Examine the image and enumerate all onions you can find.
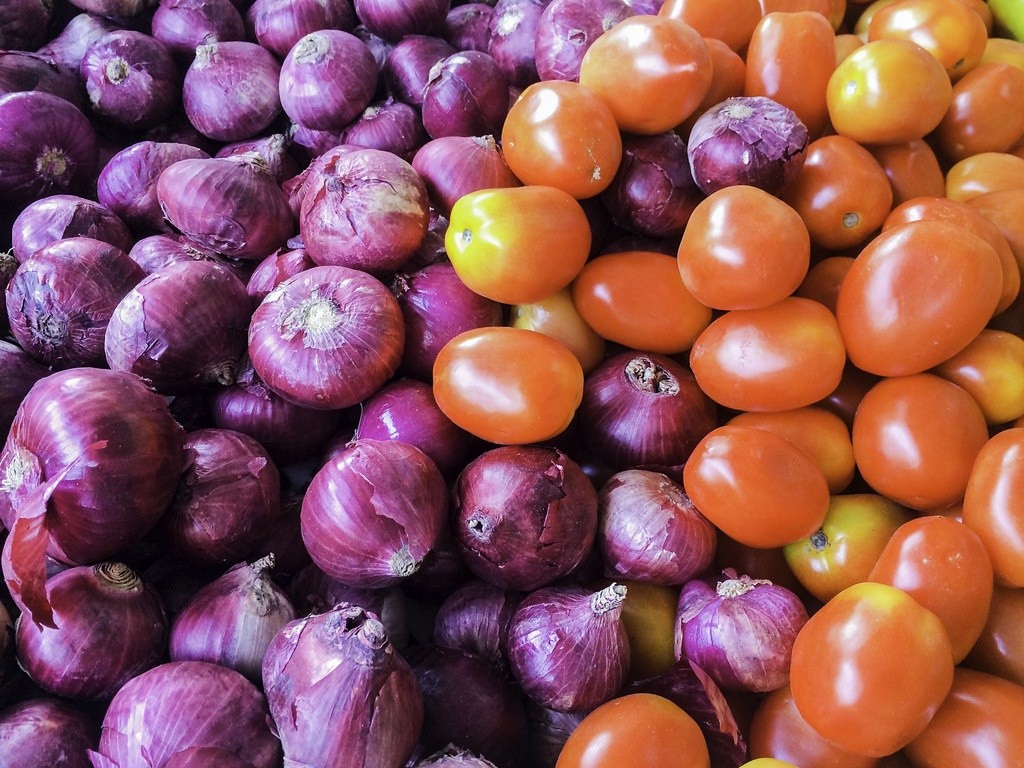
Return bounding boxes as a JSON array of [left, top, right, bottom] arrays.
[[0, 0, 811, 768]]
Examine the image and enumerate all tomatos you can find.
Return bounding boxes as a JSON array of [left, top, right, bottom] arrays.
[[431, 0, 1024, 768]]
